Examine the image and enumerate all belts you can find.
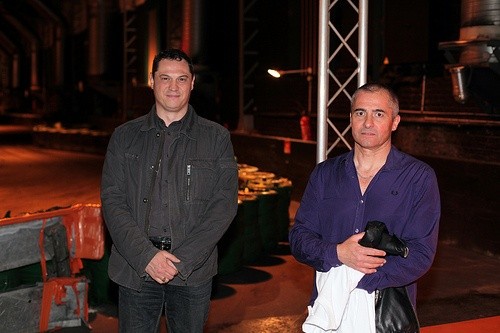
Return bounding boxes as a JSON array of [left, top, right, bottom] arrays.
[[149, 240, 172, 252]]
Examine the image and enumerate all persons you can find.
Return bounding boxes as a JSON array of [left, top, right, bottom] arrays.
[[289, 81, 441, 332], [99, 48, 240, 333]]
